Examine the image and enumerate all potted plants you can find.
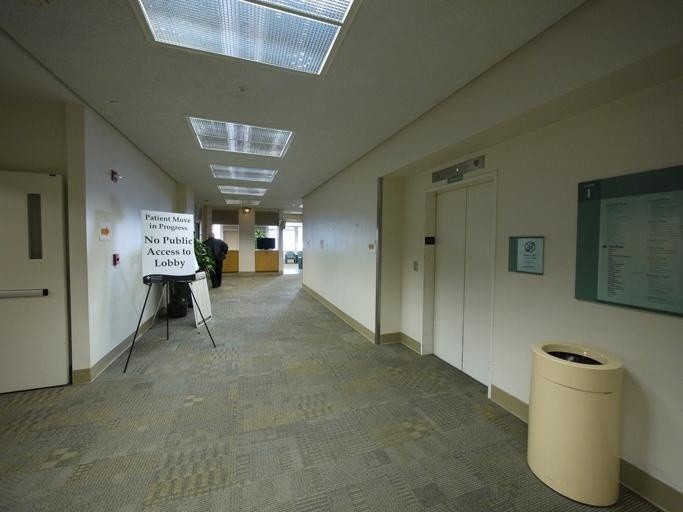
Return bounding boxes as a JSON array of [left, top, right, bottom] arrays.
[[168, 237, 215, 318]]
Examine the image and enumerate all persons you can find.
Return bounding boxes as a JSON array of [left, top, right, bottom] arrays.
[[200, 233, 228, 287]]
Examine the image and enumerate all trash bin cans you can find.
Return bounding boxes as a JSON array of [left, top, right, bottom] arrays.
[[527, 341, 623, 506]]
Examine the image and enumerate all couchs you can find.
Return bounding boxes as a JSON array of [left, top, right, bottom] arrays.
[[284, 251, 296, 264], [295, 250, 302, 263]]
